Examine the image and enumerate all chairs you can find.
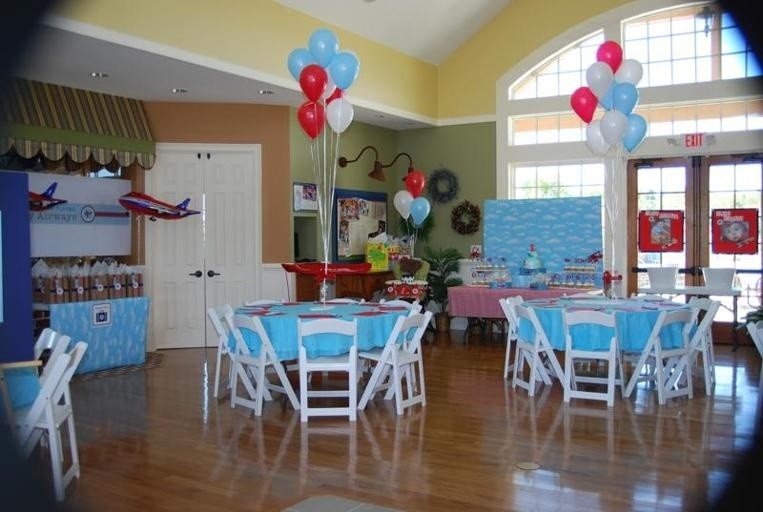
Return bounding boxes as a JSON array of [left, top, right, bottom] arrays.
[[0, 325, 89, 503], [207, 256, 432, 423], [746, 320, 763, 395], [498, 294, 721, 407]]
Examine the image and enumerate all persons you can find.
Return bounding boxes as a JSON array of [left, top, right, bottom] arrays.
[[338, 198, 369, 243], [302, 184, 316, 201]]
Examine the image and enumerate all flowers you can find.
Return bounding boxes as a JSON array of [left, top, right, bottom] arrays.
[[449, 199, 480, 234]]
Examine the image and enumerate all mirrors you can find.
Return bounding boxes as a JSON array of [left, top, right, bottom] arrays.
[[293, 214, 320, 263]]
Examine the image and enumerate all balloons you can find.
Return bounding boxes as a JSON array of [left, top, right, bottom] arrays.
[[393, 190, 414, 219], [405, 170, 425, 198], [410, 197, 430, 225], [569, 41, 648, 158], [286, 27, 360, 139]]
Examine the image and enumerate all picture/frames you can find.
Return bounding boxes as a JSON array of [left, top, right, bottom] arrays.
[[712, 209, 758, 255], [638, 210, 684, 253]]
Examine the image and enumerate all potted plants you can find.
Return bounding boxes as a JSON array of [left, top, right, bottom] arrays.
[[422, 245, 467, 332]]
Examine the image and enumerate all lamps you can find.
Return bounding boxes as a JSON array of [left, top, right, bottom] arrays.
[[381, 153, 414, 181], [339, 145, 385, 181]]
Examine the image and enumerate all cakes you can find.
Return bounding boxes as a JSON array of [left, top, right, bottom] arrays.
[[522, 244, 542, 269]]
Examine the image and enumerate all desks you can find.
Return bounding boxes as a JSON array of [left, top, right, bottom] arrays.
[[447, 285, 603, 345], [639, 286, 742, 352]]
